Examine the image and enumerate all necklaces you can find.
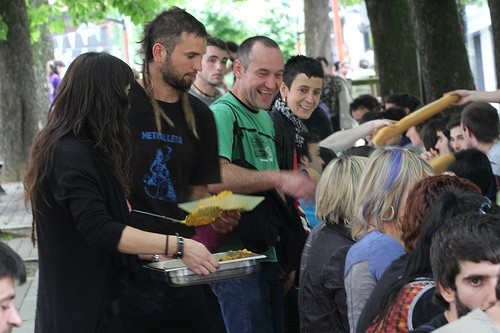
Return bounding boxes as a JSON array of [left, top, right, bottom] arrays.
[[192, 81, 217, 99]]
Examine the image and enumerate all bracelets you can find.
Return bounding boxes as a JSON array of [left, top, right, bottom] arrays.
[[164, 234, 169, 255], [172, 236, 184, 259], [152, 253, 159, 262]]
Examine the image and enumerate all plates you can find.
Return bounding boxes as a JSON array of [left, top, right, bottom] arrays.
[[178, 194, 265, 214]]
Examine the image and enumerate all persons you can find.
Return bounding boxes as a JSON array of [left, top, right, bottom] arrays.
[[190, 38, 239, 106], [22, 52, 218, 333], [46, 60, 65, 103], [208, 36, 316, 333], [129, 5, 243, 333], [267, 55, 500, 333], [0, 242, 27, 333]]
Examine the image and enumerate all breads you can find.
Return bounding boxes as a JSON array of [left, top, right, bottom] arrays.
[[373, 95, 457, 144], [426, 152, 455, 172]]
